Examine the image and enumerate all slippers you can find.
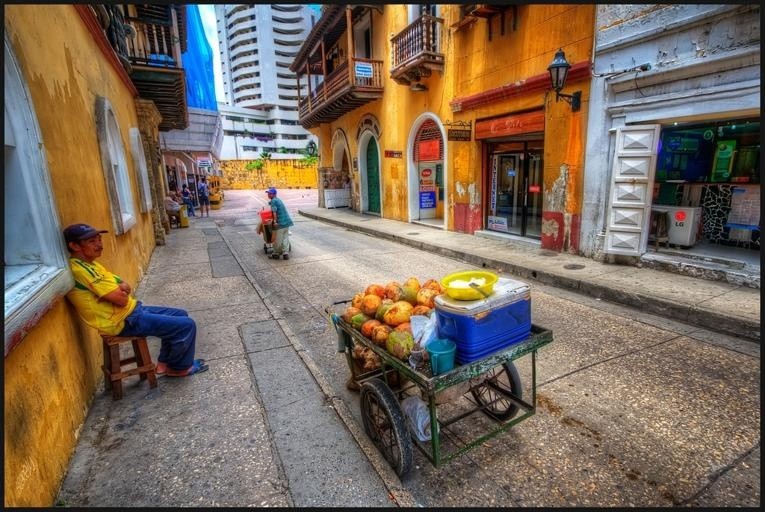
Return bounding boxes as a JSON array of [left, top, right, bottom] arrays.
[[198, 183, 207, 200]]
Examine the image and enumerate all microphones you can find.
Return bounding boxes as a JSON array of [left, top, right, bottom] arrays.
[[165, 208, 179, 228], [100, 330, 158, 402]]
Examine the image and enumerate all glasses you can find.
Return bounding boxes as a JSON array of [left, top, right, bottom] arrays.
[[409, 76, 427, 91], [545, 46, 581, 112]]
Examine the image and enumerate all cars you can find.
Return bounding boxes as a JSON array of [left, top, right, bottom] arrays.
[[427, 339, 458, 376], [409, 346, 424, 367]]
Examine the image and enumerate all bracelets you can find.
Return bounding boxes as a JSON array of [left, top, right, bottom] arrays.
[[440, 271, 498, 301]]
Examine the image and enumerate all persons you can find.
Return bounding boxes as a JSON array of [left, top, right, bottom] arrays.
[[63, 224, 210, 378], [165, 176, 210, 223], [265, 188, 294, 260]]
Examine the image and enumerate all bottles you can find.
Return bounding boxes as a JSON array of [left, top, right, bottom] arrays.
[[264, 187, 278, 195], [62, 222, 111, 244]]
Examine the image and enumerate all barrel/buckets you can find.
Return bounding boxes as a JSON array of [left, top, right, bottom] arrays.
[[154, 357, 210, 378]]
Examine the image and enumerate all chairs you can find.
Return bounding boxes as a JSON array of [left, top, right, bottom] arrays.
[[268, 254, 280, 260], [282, 254, 289, 260]]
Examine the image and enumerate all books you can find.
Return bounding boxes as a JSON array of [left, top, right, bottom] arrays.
[[432, 276, 533, 364]]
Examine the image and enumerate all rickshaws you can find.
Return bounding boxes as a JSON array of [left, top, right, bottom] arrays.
[[343, 277, 441, 358]]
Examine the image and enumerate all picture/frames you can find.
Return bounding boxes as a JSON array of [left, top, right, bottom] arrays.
[[724, 223, 759, 249]]
